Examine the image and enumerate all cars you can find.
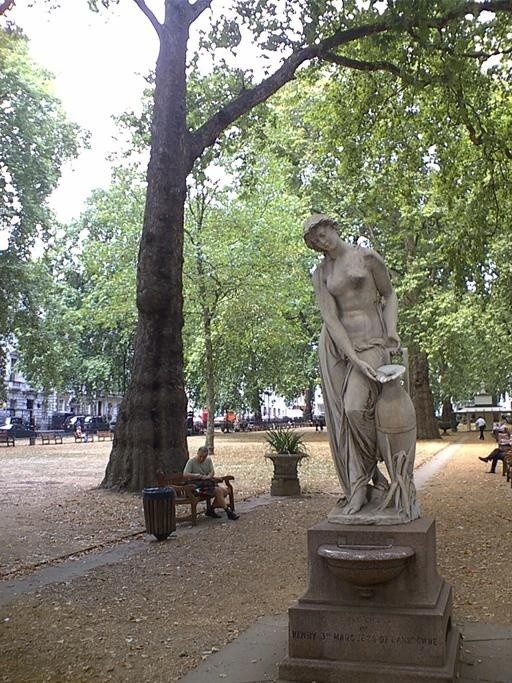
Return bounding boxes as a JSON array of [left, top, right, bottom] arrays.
[[0, 409, 109, 438]]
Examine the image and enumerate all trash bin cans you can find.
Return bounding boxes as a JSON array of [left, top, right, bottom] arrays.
[[30, 438, 35, 445], [142, 487, 176, 542]]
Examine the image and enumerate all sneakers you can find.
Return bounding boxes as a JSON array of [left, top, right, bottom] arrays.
[[205, 510, 222, 519], [478, 456, 488, 462], [485, 470, 496, 473], [227, 512, 241, 520]]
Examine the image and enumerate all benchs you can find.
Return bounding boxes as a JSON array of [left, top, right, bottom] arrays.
[[0, 430, 113, 448], [154, 469, 236, 524]]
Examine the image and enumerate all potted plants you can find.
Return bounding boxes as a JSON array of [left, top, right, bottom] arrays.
[[264, 427, 310, 496]]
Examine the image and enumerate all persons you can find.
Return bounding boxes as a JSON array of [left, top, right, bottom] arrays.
[[314, 418, 320, 432], [474, 414, 512, 473], [287, 419, 292, 425], [75, 417, 83, 431], [184, 446, 240, 520], [77, 425, 88, 442], [318, 415, 324, 430], [302, 213, 400, 517]]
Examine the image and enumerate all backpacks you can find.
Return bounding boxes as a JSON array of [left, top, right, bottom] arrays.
[[183, 479, 216, 498]]
